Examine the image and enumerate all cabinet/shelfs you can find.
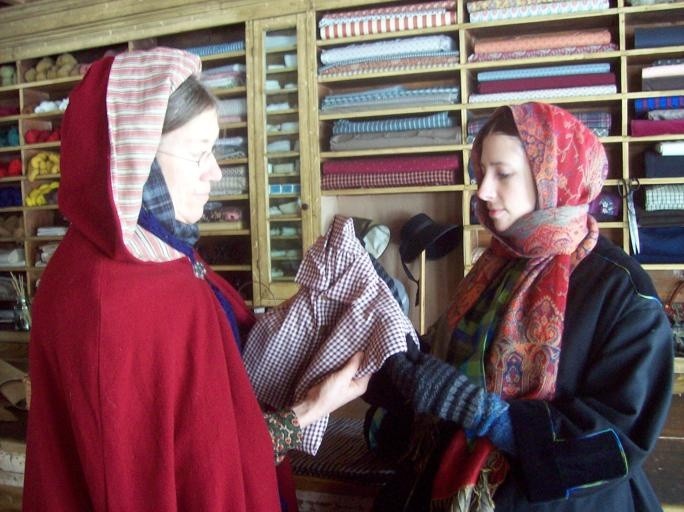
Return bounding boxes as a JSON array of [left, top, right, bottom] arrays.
[[619, 1, 683, 269], [458, 2, 627, 270], [20, 26, 133, 331], [250, 1, 318, 301], [316, 3, 466, 382], [129, 13, 256, 318], [2, 35, 30, 337]]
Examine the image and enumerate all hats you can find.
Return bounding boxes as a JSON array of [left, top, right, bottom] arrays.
[[399, 214, 459, 262]]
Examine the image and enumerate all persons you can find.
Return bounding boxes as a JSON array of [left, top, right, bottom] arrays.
[[361, 102, 674, 512], [20, 46, 371, 512]]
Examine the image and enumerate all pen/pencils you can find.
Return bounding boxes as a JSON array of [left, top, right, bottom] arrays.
[[8, 271, 32, 328]]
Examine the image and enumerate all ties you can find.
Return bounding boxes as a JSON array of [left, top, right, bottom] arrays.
[[158, 143, 216, 167]]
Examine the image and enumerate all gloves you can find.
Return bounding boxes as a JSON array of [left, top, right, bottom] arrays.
[[366, 333, 485, 429]]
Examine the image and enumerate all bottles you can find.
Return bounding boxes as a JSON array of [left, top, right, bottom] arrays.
[[14, 297, 31, 332]]
